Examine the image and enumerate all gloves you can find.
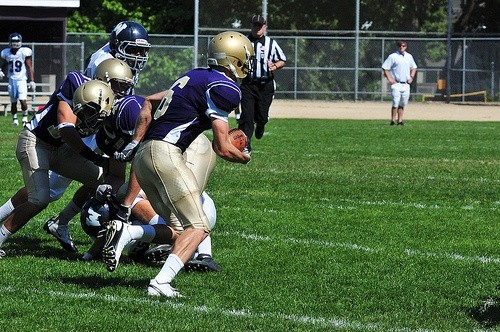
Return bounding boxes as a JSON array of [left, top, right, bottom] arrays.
[[114, 141, 139, 163], [28, 81, 36, 89], [0, 68, 5, 80]]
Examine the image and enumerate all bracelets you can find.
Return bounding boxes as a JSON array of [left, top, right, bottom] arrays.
[[411, 76, 414, 80], [275, 64, 277, 69]]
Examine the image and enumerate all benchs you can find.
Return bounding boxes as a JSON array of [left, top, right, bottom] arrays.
[[0, 101, 47, 117]]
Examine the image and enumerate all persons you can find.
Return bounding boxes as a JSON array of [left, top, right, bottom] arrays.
[[0, 33, 34, 126], [237, 15, 287, 153], [383, 41, 417, 126], [0, 22, 252, 297]]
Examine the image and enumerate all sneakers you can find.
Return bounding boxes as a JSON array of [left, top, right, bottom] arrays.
[[184, 254, 222, 273], [147, 279, 185, 297], [102, 220, 131, 272], [147, 244, 175, 265], [43, 215, 79, 253], [128, 240, 149, 256]]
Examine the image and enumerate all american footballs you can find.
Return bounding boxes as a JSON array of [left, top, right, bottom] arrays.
[[211, 128, 250, 153]]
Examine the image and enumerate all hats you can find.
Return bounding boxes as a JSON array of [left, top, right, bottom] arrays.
[[250, 14, 266, 25]]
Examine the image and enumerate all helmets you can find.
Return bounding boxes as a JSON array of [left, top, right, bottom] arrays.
[[109, 21, 148, 58], [9, 33, 22, 49], [73, 79, 115, 116], [80, 197, 117, 239], [207, 31, 254, 79], [93, 58, 134, 86]]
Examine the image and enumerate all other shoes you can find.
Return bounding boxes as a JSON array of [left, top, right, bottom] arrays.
[[254, 124, 264, 139], [242, 143, 252, 155], [22, 116, 27, 122], [390, 120, 396, 125], [398, 121, 404, 125], [13, 119, 19, 126]]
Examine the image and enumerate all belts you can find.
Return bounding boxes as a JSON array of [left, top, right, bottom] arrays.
[[396, 80, 405, 84]]
